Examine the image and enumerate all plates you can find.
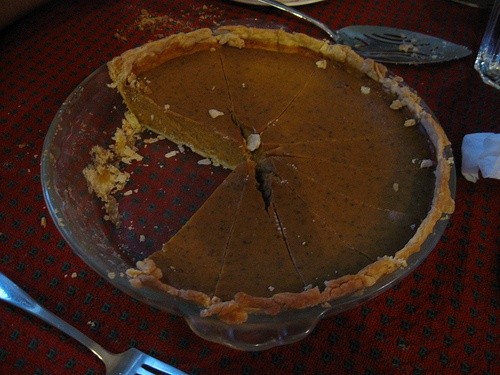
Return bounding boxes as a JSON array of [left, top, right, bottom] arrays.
[[40, 25, 457, 352]]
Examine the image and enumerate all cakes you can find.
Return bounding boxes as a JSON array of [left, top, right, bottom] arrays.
[[105, 25, 456, 324]]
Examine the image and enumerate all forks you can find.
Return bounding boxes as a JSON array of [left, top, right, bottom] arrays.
[[1, 272, 187, 375]]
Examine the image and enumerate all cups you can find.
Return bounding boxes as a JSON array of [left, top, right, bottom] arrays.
[[474, 0, 500, 86]]
